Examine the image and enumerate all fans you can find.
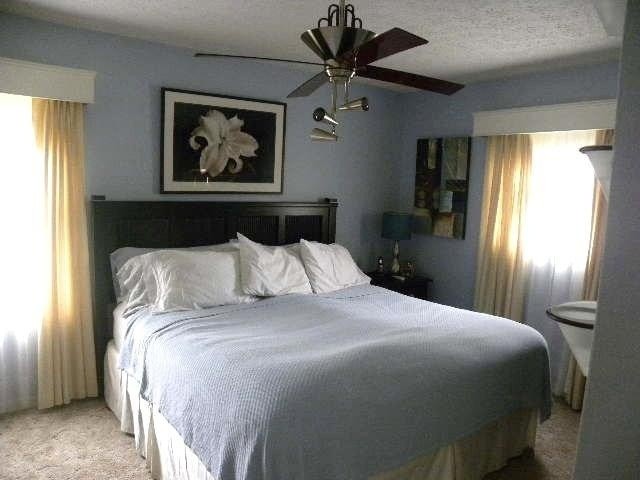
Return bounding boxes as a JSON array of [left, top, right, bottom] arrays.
[[192, 1, 466, 99]]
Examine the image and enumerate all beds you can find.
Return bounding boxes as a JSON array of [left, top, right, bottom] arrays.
[[87, 194, 550, 480]]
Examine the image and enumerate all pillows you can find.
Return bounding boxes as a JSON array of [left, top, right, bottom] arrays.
[[148, 248, 249, 315], [298, 237, 373, 296], [236, 231, 313, 296]]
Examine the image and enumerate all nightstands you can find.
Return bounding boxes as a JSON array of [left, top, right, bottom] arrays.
[[364, 272, 433, 301]]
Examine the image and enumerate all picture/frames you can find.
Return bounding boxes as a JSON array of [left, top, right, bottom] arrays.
[[158, 86, 288, 196], [413, 136, 473, 242]]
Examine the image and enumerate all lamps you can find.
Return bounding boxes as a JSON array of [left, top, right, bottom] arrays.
[[381, 211, 416, 279], [300, 67, 379, 143]]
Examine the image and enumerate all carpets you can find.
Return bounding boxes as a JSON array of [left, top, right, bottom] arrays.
[[0, 394, 157, 480], [484, 390, 583, 480]]
